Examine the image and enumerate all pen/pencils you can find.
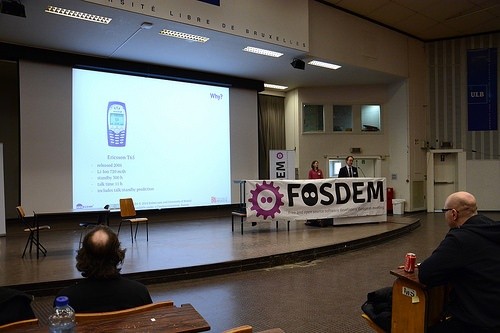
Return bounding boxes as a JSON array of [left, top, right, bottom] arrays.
[[398, 264, 418, 268]]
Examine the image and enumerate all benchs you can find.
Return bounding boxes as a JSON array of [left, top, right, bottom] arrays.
[[0, 300, 175, 329]]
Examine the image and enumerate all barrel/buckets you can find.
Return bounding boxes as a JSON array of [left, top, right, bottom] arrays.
[[392, 199, 406, 214]]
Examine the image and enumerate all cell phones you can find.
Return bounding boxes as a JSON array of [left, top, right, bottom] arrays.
[[107, 101, 127, 147]]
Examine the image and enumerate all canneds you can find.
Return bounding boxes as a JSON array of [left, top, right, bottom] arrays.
[[404, 253, 416, 273]]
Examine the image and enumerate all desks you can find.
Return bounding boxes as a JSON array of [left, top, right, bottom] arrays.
[[29, 209, 110, 260], [232, 178, 387, 235], [390, 266, 454, 333], [0, 303, 211, 333]]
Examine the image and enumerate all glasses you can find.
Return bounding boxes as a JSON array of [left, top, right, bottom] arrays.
[[442, 208, 459, 213]]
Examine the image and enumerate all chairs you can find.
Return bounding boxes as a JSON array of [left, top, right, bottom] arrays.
[[79, 205, 111, 250], [117, 198, 149, 243], [15, 206, 51, 261]]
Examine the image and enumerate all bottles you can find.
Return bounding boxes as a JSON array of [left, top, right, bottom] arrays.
[[48, 296, 75, 333]]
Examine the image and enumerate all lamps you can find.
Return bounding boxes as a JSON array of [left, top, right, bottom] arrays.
[[0, 0, 26, 18], [290, 57, 306, 70]]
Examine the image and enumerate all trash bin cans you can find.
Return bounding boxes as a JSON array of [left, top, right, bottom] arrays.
[[392, 198, 405, 214]]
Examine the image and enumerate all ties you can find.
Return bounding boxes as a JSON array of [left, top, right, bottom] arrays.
[[349, 166, 352, 177]]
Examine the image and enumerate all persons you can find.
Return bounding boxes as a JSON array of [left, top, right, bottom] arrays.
[[53, 225, 153, 313], [338, 156, 358, 177], [309, 160, 324, 179], [417, 192, 500, 333]]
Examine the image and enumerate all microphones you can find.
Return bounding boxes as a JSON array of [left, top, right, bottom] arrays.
[[358, 167, 365, 177]]
